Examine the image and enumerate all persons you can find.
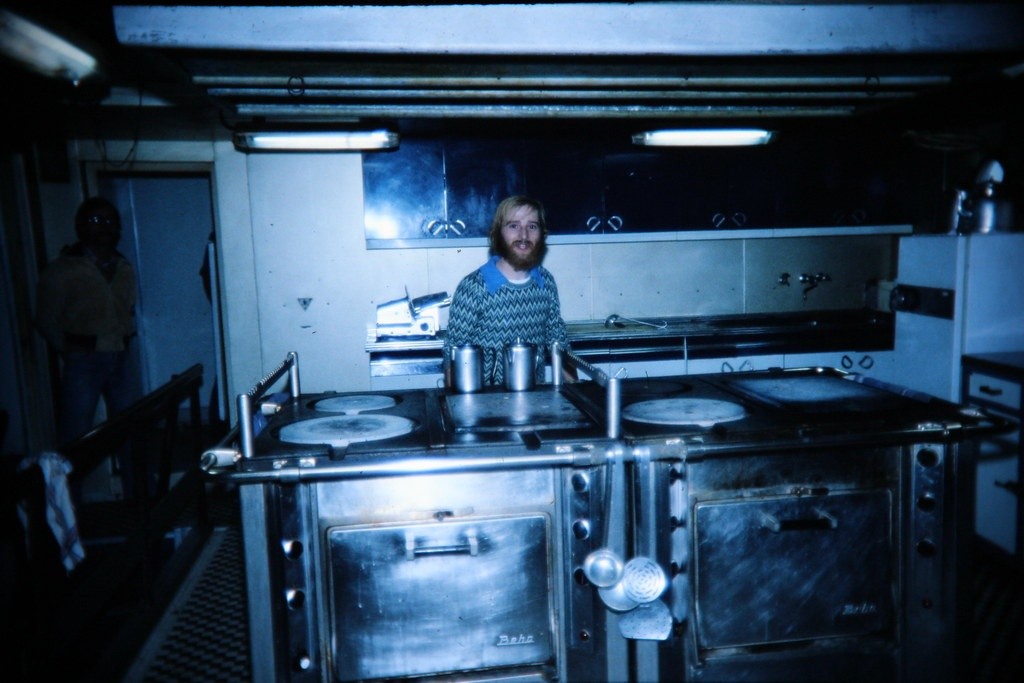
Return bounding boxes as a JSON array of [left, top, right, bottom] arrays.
[[36, 196, 153, 493], [444, 195, 580, 386]]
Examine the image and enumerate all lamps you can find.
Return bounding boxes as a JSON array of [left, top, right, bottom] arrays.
[[629, 124, 773, 147], [233, 128, 399, 152]]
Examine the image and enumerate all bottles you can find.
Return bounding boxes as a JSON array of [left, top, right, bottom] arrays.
[[978, 183, 995, 234]]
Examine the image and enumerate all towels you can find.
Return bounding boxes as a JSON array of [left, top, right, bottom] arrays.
[[40, 451, 86, 576]]
